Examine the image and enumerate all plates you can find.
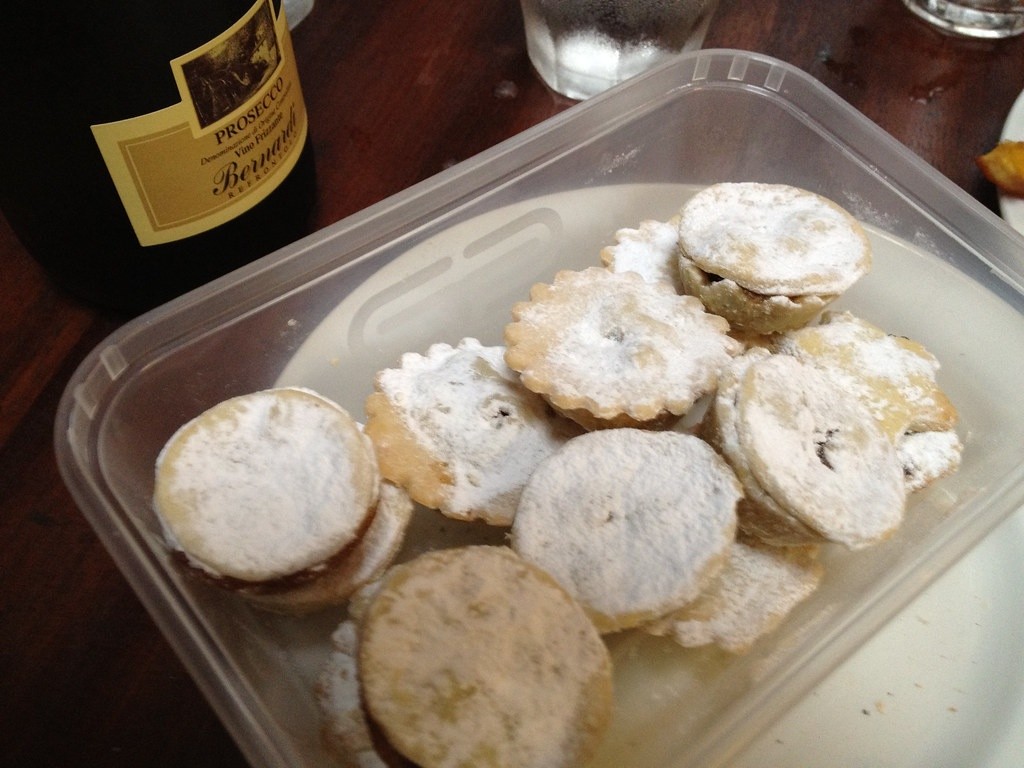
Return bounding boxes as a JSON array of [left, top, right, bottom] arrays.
[[264, 178, 1024, 768], [998, 86, 1024, 233]]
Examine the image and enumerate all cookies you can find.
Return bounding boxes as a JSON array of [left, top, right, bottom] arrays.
[[151, 181, 963, 768]]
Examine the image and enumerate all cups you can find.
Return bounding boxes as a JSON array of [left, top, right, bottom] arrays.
[[519, 0, 715, 103]]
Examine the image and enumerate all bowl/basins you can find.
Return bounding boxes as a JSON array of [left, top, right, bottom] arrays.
[[56, 43, 1024, 768]]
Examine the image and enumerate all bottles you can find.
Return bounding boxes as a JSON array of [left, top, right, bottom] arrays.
[[0, 0, 319, 315]]
